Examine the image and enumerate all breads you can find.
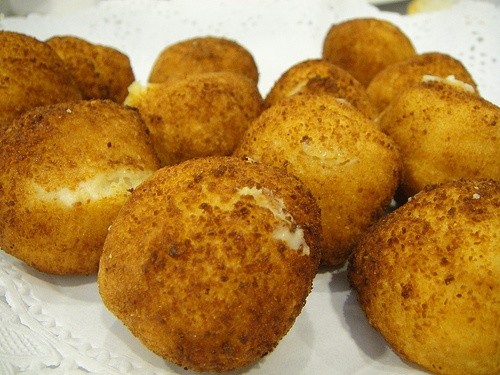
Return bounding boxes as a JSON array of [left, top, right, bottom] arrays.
[[1, 15, 500, 375]]
